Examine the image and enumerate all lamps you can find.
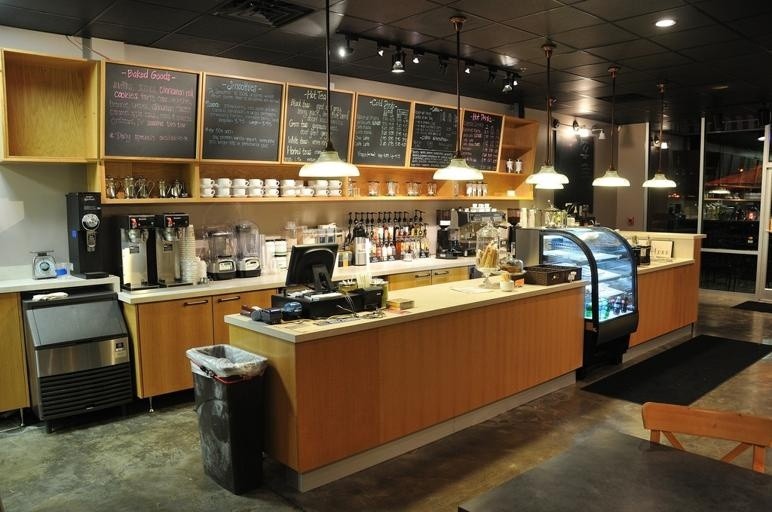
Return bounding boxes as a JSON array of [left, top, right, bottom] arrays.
[[640, 82, 677, 189], [524, 41, 570, 185], [298, 1, 361, 179], [339, 32, 519, 96], [591, 66, 631, 188], [431, 16, 485, 181], [707, 135, 731, 195]]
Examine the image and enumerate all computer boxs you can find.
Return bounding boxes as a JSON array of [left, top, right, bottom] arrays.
[[271, 288, 364, 318]]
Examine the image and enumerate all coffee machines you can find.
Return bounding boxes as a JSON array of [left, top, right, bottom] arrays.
[[117, 213, 159, 291], [159, 210, 191, 287], [204, 223, 237, 281], [64, 191, 111, 280], [434, 208, 476, 259]]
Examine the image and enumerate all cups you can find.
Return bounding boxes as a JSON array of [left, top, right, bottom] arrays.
[[506, 159, 523, 174], [468, 203, 490, 212], [466, 181, 488, 197], [339, 282, 357, 291], [519, 208, 535, 228], [500, 280, 515, 291], [349, 180, 437, 198], [182, 225, 196, 280], [200, 178, 343, 198], [370, 283, 390, 309]]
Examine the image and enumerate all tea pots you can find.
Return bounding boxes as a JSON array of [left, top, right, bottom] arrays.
[[106, 175, 185, 198]]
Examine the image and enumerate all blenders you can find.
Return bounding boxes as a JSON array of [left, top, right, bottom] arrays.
[[236, 220, 262, 276]]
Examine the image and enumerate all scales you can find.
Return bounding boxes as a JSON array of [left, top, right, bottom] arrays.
[[30, 250, 57, 279]]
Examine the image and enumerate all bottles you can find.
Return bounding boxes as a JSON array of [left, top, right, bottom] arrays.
[[344, 208, 429, 266]]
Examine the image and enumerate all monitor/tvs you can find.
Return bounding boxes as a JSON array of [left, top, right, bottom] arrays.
[[285, 242, 338, 293]]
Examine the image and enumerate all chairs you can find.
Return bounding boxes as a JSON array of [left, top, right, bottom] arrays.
[[641, 401, 772, 473]]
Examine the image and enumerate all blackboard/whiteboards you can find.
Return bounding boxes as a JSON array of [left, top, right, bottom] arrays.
[[352, 94, 411, 167], [105, 62, 198, 160], [460, 110, 503, 172], [284, 83, 353, 165], [410, 102, 461, 170], [202, 74, 284, 163]]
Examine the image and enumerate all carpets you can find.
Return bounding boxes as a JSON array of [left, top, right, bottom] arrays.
[[579, 334, 772, 408], [730, 300, 771, 313]]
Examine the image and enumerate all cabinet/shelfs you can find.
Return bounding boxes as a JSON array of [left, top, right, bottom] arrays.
[[198, 160, 348, 201], [0, 291, 30, 413], [1, 49, 101, 164], [87, 158, 198, 203], [454, 171, 533, 202], [499, 114, 540, 177], [673, 150, 761, 190], [123, 288, 278, 398], [388, 266, 472, 292], [348, 166, 453, 199]]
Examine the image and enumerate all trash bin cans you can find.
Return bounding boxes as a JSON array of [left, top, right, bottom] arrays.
[[186, 344, 268, 495]]
